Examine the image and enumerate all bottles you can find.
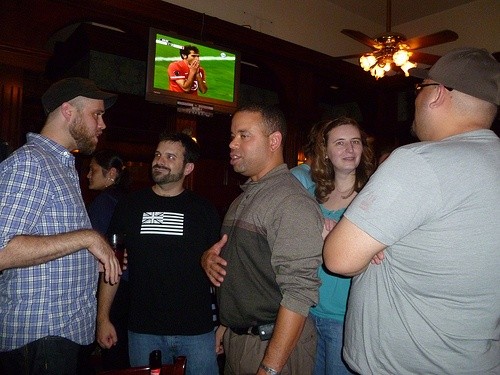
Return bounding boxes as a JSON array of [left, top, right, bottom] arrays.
[[149, 350, 162, 375]]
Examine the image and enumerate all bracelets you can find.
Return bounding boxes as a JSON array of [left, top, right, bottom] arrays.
[[201, 103, 324, 375]]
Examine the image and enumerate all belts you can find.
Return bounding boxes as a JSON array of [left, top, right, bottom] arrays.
[[229, 325, 259, 335]]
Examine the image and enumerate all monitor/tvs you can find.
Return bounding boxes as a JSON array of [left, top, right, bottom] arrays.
[[145, 27, 241, 115]]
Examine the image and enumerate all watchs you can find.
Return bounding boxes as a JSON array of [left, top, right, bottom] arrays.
[[260, 364, 280, 375]]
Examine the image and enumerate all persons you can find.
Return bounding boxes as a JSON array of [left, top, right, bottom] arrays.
[[0, 77, 122, 375], [168, 46, 207, 95], [97, 132, 221, 375], [87, 151, 135, 369], [306, 117, 374, 375], [289, 121, 332, 198], [323, 48, 500, 375]]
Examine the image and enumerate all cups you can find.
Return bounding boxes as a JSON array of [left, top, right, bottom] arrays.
[[107, 233, 125, 273]]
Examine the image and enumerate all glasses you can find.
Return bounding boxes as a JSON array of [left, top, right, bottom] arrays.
[[414, 83, 453, 96]]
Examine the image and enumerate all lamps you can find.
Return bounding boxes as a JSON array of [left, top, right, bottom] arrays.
[[358, 34, 418, 80]]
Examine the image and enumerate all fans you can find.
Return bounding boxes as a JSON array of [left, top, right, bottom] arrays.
[[340, 0, 459, 65]]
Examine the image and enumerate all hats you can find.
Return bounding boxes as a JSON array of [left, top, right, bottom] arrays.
[[41, 78, 117, 119], [408, 48, 500, 105]]
